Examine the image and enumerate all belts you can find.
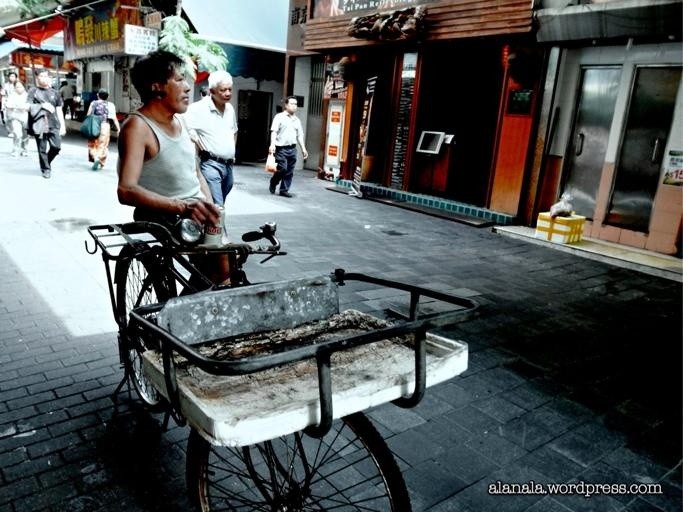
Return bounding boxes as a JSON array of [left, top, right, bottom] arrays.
[[210, 156, 234, 165]]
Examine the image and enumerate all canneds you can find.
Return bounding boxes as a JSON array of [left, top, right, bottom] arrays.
[[203, 203, 226, 245]]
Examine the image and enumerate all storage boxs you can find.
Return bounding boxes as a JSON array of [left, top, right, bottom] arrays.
[[534, 212, 585, 244]]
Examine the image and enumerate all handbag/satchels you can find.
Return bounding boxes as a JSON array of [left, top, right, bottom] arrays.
[[80, 101, 101, 137]]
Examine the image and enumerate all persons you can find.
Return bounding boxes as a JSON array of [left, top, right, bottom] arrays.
[[87, 89, 120, 169], [0, 68, 74, 179], [269, 96, 308, 197], [180, 70, 238, 236], [118, 52, 231, 297]]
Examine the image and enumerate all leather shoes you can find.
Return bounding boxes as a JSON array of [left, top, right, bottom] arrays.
[[269, 182, 292, 197], [43, 168, 50, 178]]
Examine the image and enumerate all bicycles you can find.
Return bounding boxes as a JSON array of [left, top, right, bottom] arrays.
[[83, 210, 483, 511]]
